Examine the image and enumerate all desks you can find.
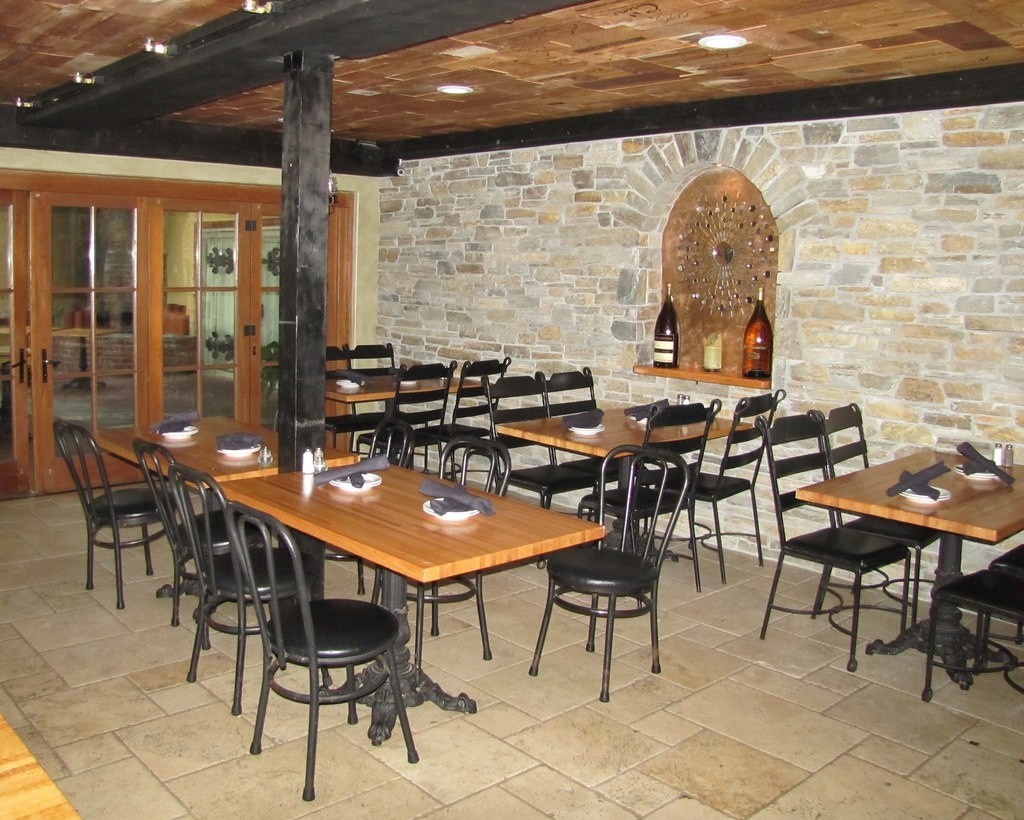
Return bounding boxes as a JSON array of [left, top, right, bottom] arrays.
[[92, 414, 361, 511], [494, 405, 753, 552], [216, 464, 606, 748], [795, 449, 1024, 691], [326, 374, 494, 465], [53, 328, 118, 391], [0, 324, 30, 410]]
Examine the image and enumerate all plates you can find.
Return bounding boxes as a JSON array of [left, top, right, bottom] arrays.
[[954, 463, 1001, 480], [900, 485, 951, 503], [629, 414, 649, 423], [993, 442, 1015, 467], [396, 381, 418, 384], [423, 497, 480, 521], [157, 426, 199, 438], [568, 423, 605, 435], [216, 444, 261, 458], [329, 472, 382, 493], [336, 379, 365, 387]]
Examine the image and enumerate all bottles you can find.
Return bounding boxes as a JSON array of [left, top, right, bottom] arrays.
[[652, 283, 678, 367], [742, 287, 773, 378], [302, 448, 326, 474], [258, 446, 273, 466]]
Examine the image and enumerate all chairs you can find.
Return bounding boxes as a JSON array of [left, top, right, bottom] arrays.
[[52, 420, 177, 610], [225, 500, 420, 803], [132, 439, 265, 650], [324, 338, 1023, 703], [168, 465, 319, 718]]
